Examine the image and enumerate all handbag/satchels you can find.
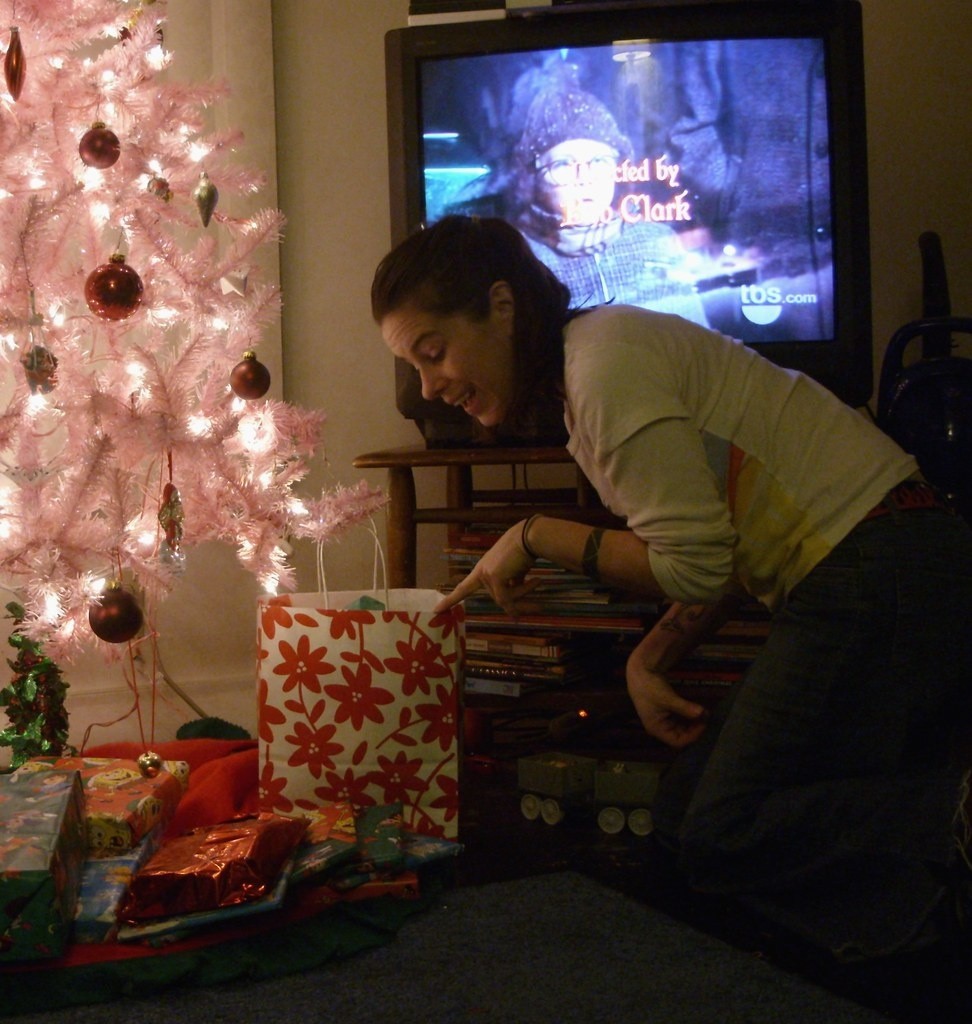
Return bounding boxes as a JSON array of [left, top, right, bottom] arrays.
[[256, 514, 465, 850]]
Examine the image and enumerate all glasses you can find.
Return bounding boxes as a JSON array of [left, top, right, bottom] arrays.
[[535, 157, 616, 185]]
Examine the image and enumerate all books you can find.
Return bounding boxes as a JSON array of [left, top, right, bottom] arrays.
[[436, 520, 772, 700]]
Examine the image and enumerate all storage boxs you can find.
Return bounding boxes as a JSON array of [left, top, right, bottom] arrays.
[[0, 771, 92, 964], [13, 752, 190, 854], [66, 826, 161, 947], [517, 752, 598, 798], [592, 761, 669, 805]]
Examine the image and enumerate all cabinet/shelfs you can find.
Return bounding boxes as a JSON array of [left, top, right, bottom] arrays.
[[354, 444, 769, 884]]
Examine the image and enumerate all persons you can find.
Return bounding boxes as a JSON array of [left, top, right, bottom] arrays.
[[367, 212, 971, 833], [491, 67, 705, 330]]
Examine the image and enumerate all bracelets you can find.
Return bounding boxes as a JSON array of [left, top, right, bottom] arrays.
[[521, 513, 544, 559]]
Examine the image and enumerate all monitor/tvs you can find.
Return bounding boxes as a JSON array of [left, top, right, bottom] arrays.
[[386, 0, 875, 445]]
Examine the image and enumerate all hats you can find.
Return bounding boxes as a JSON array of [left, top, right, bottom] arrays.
[[514, 86, 631, 169]]
